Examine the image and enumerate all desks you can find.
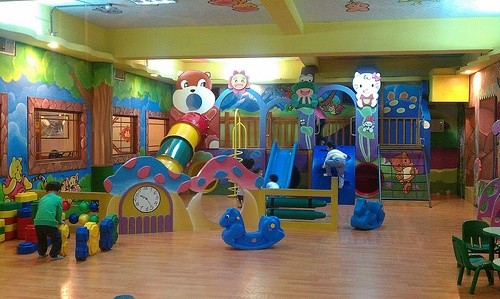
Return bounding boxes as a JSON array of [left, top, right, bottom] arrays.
[[482, 226, 500, 261]]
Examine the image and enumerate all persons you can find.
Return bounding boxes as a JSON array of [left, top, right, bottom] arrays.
[[35, 182, 65, 260], [266, 174, 279, 201], [321, 141, 347, 189], [237, 167, 262, 203]]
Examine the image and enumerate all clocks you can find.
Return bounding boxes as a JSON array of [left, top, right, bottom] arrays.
[[133, 185, 162, 214]]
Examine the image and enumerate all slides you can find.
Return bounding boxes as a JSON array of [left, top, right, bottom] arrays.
[[262, 140, 298, 189], [153, 111, 209, 174]]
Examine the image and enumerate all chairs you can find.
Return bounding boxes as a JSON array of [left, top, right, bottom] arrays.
[[452, 234, 494, 294], [461, 219, 496, 259], [492, 257, 500, 271]]
